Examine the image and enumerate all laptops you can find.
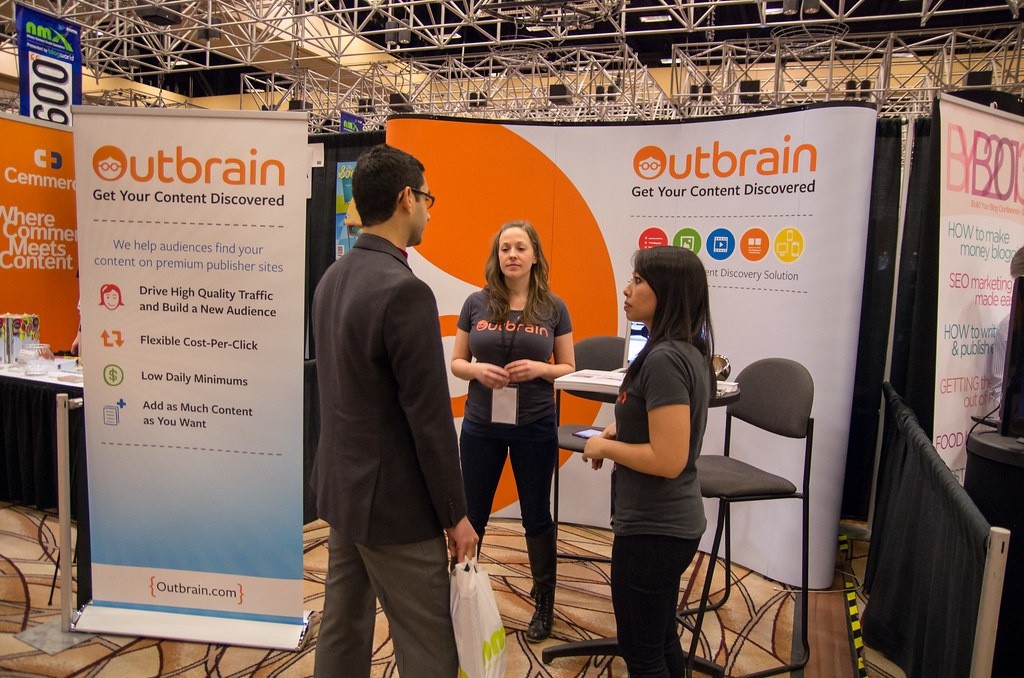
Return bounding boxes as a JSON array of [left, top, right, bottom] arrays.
[[610, 319, 708, 380]]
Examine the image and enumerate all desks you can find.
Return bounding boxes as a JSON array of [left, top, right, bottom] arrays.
[[0, 355, 88, 518], [543, 380, 741, 678]]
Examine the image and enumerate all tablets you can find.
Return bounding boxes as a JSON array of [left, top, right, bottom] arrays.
[[571, 428, 605, 439]]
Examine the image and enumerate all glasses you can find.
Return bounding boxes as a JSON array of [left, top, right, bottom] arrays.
[[398, 187, 435, 209]]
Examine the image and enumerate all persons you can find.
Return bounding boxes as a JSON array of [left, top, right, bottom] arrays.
[[70, 270, 81, 357], [449, 220, 576, 643], [310, 144, 479, 678], [580, 245, 717, 678]]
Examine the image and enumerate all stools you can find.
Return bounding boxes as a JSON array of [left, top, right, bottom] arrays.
[[557, 336, 815, 678]]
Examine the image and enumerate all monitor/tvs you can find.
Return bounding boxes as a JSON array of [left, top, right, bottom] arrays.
[[996, 275, 1024, 444]]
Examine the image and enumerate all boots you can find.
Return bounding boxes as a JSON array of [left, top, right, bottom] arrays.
[[524, 523, 557, 641]]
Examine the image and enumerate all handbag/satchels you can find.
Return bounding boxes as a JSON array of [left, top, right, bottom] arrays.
[[449, 545, 507, 678]]
